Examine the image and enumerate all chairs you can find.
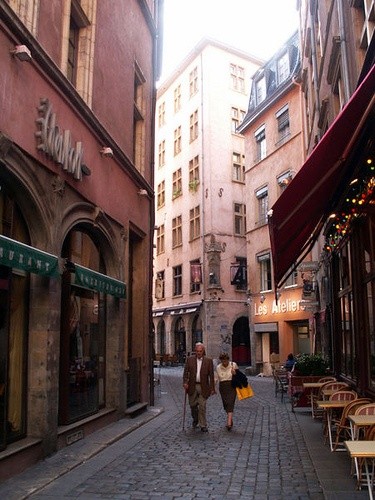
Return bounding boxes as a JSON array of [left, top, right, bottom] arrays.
[[271, 364, 375, 500]]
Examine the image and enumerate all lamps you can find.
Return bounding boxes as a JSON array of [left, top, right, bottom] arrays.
[[99, 146, 113, 158], [260, 294, 266, 304], [9, 44, 31, 62], [136, 188, 148, 199], [209, 271, 216, 284], [246, 296, 253, 306], [275, 292, 281, 300]]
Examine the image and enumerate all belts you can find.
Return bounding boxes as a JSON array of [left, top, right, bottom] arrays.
[[195, 382, 200, 384]]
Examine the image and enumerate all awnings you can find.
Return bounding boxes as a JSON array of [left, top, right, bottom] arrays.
[[267, 67, 375, 305], [0, 235, 127, 300]]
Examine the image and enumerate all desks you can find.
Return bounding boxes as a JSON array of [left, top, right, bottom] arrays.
[[288, 376, 318, 411], [344, 441, 375, 491], [322, 390, 334, 400], [302, 382, 325, 419], [316, 400, 354, 452], [348, 415, 375, 440]]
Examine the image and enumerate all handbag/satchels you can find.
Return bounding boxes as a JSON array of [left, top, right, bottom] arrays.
[[236, 384, 254, 401]]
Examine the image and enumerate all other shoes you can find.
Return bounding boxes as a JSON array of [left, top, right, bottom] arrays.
[[225, 421, 233, 431], [201, 427, 208, 432], [192, 421, 198, 426]]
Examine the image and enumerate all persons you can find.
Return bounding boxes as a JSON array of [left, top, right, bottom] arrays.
[[182, 343, 215, 433], [215, 352, 240, 430], [284, 355, 297, 372]]
